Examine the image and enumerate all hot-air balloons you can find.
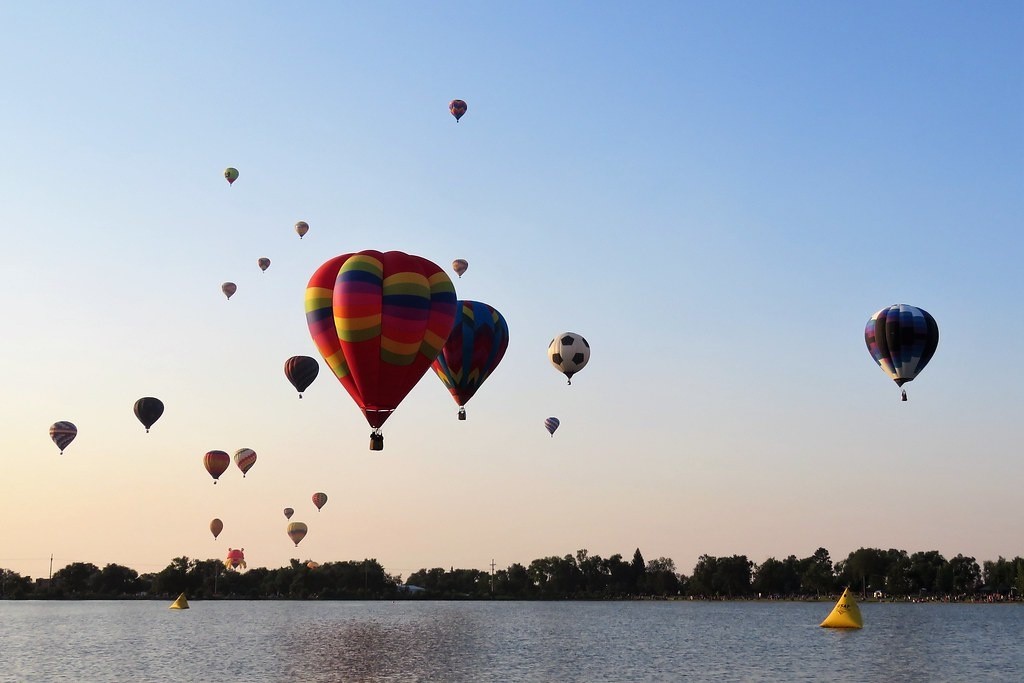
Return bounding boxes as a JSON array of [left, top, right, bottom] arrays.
[[210, 519, 223, 540], [284, 508, 294, 520], [453, 259, 468, 278], [224, 167, 239, 185], [222, 283, 237, 301], [284, 356, 319, 399], [202, 450, 230, 484], [545, 417, 560, 438], [312, 493, 327, 512], [295, 221, 309, 239], [234, 448, 257, 477], [548, 332, 590, 385], [449, 99, 467, 124], [864, 303, 941, 402], [429, 300, 510, 420], [305, 249, 457, 450], [133, 397, 164, 432], [258, 258, 271, 273], [49, 421, 78, 455], [287, 522, 307, 547]]
